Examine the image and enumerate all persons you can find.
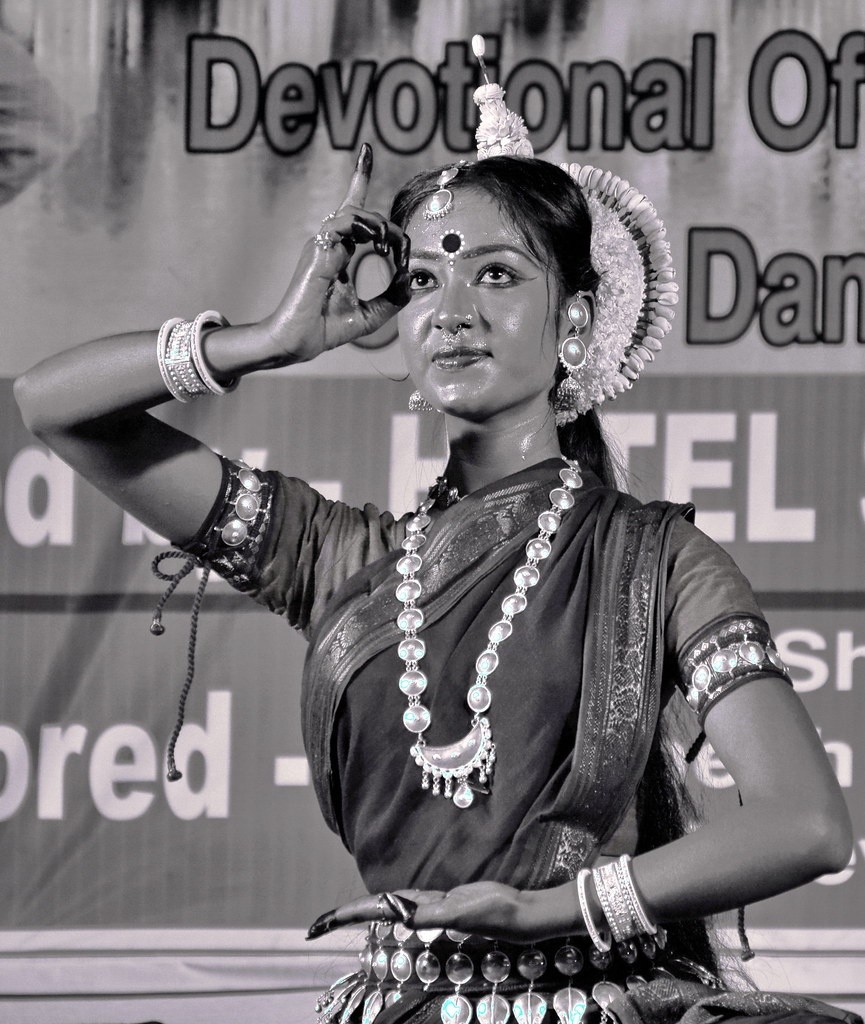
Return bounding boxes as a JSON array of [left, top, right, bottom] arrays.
[[12, 143, 858, 1024]]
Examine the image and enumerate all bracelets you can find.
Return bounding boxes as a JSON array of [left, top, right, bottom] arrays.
[[157, 309, 241, 404], [578, 852, 657, 952]]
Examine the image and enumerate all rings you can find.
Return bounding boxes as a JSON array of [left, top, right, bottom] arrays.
[[377, 896, 395, 926], [313, 231, 333, 250], [321, 210, 337, 226]]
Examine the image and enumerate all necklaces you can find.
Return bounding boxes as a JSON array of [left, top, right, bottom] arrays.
[[396, 459, 583, 809]]
